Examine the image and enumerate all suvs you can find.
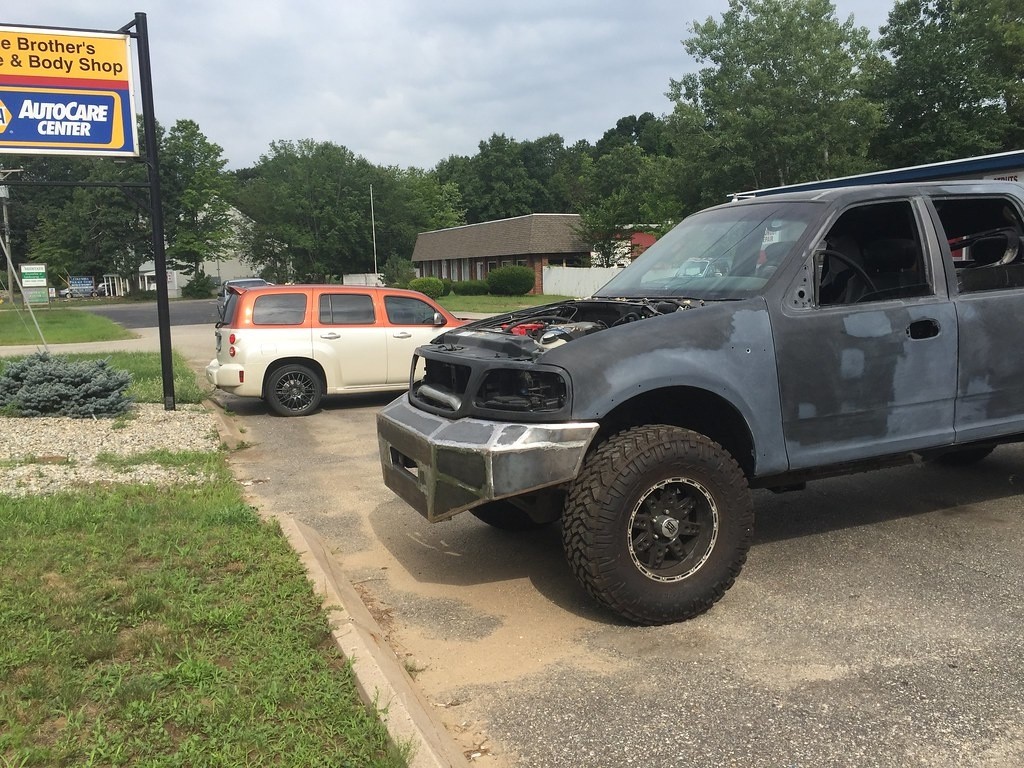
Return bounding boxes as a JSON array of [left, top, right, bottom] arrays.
[[375, 180, 1024, 625]]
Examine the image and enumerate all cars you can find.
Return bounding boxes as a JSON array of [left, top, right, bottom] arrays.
[[218, 279, 273, 307], [59, 287, 99, 298]]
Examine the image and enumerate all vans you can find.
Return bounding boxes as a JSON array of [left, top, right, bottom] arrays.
[[96, 282, 115, 296], [204, 282, 481, 417]]
